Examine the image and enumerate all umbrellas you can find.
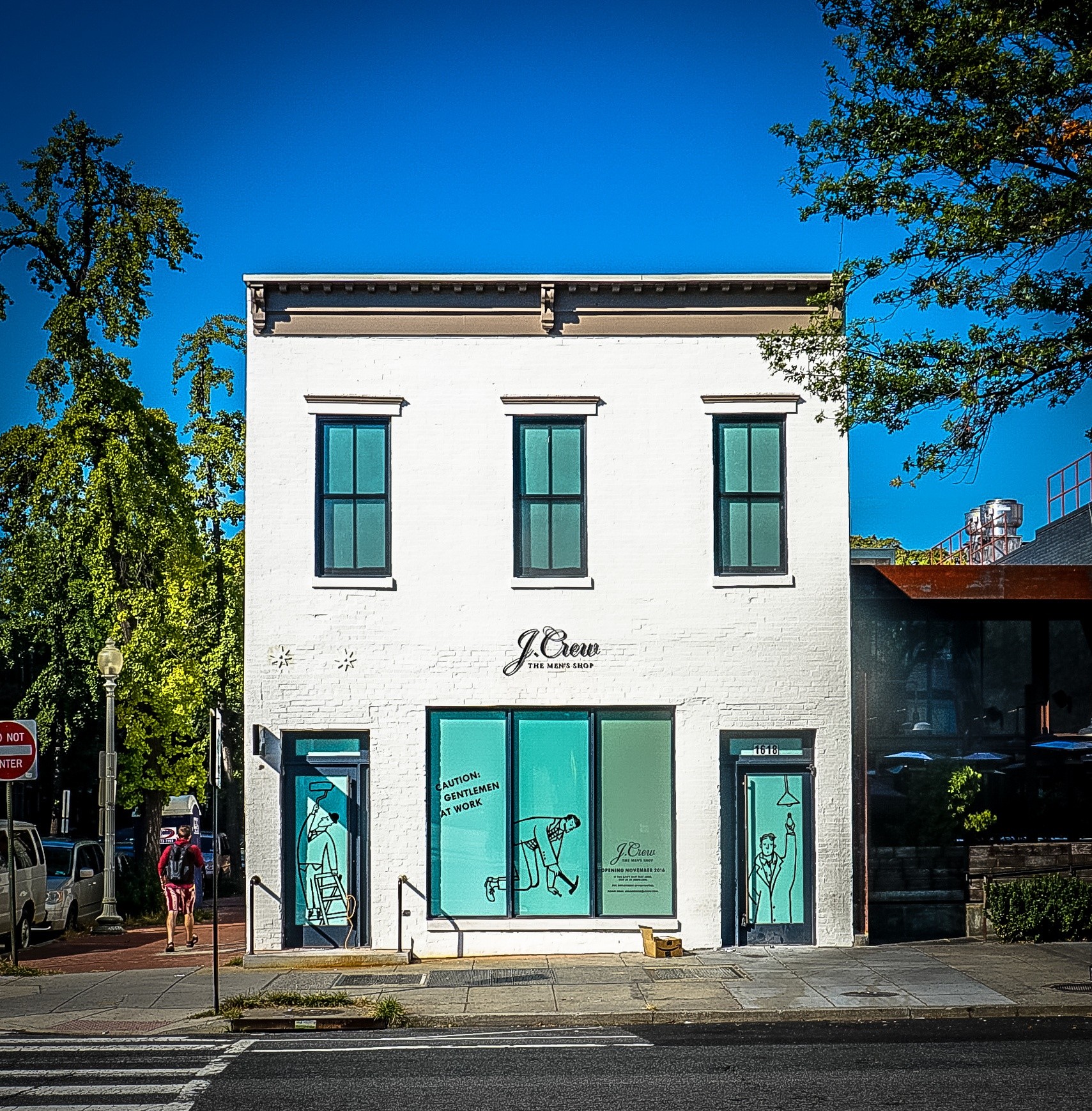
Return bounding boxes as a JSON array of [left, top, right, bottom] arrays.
[[1033, 739, 1092, 752], [885, 750, 943, 764], [960, 751, 1011, 763]]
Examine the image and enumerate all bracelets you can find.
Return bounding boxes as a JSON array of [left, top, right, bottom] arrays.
[[160, 881, 165, 883]]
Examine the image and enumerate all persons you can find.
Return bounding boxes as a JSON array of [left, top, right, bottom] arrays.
[[158, 825, 204, 952]]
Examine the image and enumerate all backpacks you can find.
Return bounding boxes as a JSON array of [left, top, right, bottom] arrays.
[[161, 843, 195, 886]]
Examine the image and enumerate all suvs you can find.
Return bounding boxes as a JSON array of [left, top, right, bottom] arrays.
[[200, 832, 230, 891], [36, 837, 105, 933]]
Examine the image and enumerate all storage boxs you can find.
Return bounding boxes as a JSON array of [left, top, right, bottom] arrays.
[[637, 925, 684, 959]]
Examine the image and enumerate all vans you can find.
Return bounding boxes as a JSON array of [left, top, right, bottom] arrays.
[[0, 819, 47, 950]]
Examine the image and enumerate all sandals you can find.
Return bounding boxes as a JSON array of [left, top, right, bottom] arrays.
[[185, 933, 199, 947], [165, 942, 175, 952]]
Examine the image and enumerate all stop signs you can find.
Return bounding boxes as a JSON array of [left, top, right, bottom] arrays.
[[0, 720, 38, 781]]
